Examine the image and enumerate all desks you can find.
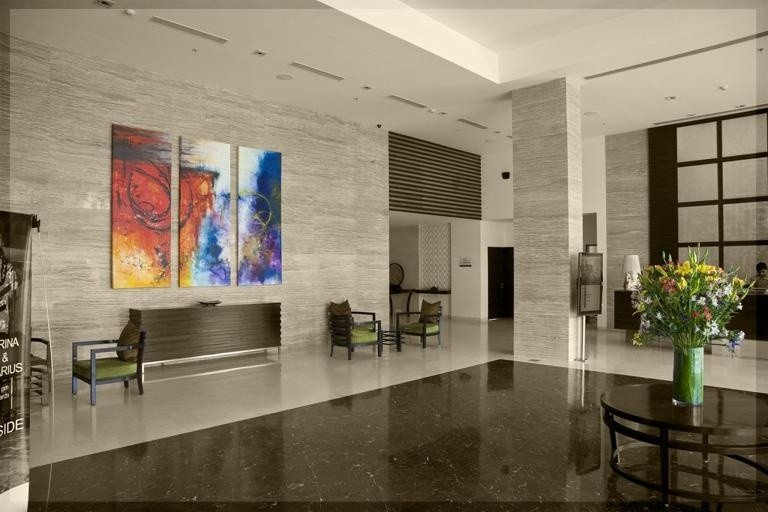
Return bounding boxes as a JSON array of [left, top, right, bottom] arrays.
[[601, 384, 768, 507]]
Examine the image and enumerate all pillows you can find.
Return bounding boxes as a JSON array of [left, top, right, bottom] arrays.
[[330, 299, 353, 330], [419, 299, 441, 324], [116, 321, 141, 362]]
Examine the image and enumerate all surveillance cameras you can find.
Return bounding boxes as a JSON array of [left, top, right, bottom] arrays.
[[502, 171, 510, 179]]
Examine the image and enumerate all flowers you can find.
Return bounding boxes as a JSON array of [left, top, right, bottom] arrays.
[[630, 240, 759, 404]]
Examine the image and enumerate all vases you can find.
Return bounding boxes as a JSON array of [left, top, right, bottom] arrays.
[[672, 343, 704, 407]]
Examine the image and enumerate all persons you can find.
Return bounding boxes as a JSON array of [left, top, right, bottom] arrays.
[[750, 262, 768, 288]]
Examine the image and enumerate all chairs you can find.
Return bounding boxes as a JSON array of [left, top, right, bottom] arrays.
[[328, 306, 443, 360], [72, 331, 146, 404], [30, 336, 50, 406]]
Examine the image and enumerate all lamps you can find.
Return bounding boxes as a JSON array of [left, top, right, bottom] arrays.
[[622, 255, 641, 290]]
